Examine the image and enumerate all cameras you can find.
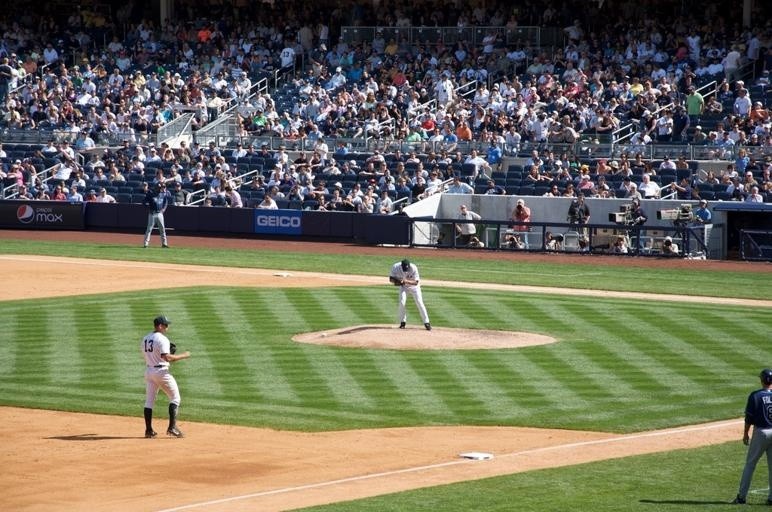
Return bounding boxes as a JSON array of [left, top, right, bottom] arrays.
[[665, 240, 671, 246], [517, 205, 522, 210], [510, 237, 514, 241]]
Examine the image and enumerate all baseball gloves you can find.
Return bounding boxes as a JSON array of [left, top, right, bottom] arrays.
[[170, 343, 176, 354], [390, 276, 403, 286]]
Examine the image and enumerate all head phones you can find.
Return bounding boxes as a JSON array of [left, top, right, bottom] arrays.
[[632, 198, 640, 206], [699, 199, 708, 207]]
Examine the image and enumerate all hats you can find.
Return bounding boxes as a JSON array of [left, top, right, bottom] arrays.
[[335, 66, 341, 71], [320, 43, 327, 50], [153, 315, 171, 327], [401, 259, 410, 271], [7, 71, 761, 207]]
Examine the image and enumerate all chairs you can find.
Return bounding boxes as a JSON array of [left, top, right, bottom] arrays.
[[0, 141, 772, 214]]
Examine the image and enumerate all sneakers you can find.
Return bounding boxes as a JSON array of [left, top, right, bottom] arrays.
[[166, 427, 181, 437], [144, 429, 158, 438], [423, 323, 431, 330], [399, 322, 405, 328], [732, 493, 746, 504]]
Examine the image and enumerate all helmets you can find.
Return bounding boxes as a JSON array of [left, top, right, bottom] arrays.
[[758, 368, 771, 385]]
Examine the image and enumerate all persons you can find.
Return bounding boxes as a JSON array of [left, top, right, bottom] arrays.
[[730, 368, 772, 506], [390, 259, 432, 330], [142, 316, 191, 437]]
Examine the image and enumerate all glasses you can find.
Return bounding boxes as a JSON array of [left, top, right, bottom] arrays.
[[162, 324, 169, 328]]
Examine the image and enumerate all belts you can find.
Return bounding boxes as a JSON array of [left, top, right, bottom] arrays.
[[152, 364, 166, 369]]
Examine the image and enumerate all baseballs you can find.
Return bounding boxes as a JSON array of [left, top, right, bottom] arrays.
[[321, 333, 326, 337]]
